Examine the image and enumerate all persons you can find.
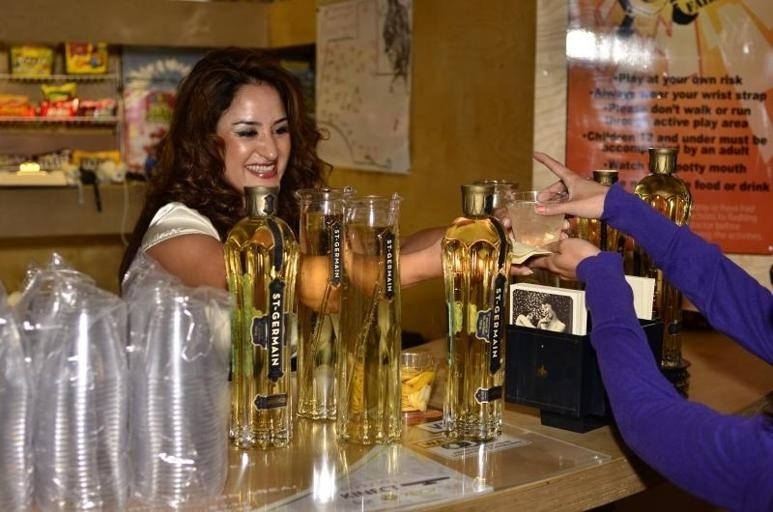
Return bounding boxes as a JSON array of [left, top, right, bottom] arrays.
[[111, 48, 570, 381], [537, 297, 566, 331], [531, 150, 772, 512]]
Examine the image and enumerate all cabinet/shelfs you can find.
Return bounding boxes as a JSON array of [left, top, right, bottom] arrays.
[[0, 72, 118, 129]]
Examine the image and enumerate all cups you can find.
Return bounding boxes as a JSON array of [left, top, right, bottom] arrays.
[[1, 267, 217, 512], [499, 187, 566, 259], [336, 191, 406, 447], [292, 182, 353, 428], [398, 349, 439, 411]]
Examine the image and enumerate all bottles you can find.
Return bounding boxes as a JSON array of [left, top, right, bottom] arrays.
[[437, 180, 515, 444], [571, 163, 633, 268], [631, 145, 694, 373], [224, 183, 296, 447]]
